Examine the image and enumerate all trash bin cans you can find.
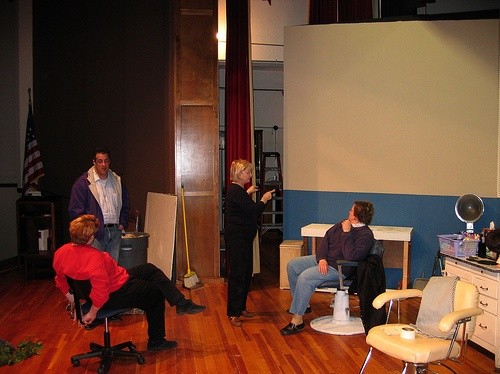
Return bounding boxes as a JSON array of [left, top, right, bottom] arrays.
[[119, 231, 150, 271]]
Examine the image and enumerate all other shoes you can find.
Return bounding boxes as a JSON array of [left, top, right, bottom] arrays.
[[85, 318, 104, 329], [107, 315, 122, 321], [240, 311, 254, 317], [230, 316, 241, 327]]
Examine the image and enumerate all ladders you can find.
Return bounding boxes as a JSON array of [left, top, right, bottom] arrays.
[[258, 151, 284, 240]]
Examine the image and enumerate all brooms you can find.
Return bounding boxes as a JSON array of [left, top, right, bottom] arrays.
[[180, 183, 205, 290]]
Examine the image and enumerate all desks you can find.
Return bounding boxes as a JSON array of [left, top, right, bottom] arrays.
[[301, 221, 413, 296], [17, 198, 58, 276], [280, 239, 307, 290]]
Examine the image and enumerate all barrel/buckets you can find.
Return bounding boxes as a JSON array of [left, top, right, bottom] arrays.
[[118, 231, 150, 269]]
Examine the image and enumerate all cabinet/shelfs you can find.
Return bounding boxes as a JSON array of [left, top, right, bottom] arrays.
[[441, 254, 500, 371], [260, 153, 283, 232]]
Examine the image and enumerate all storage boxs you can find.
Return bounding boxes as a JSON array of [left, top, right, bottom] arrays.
[[440, 235, 479, 260]]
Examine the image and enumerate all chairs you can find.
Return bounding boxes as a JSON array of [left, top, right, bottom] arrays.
[[64, 275, 145, 374], [357, 274, 484, 374], [309, 241, 384, 335]]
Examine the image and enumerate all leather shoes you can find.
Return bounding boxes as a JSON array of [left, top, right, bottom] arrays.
[[287, 305, 311, 314], [280, 321, 304, 335], [176, 299, 206, 314], [147, 338, 176, 350]]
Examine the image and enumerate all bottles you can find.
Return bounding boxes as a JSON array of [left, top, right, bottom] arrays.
[[478, 229, 487, 258]]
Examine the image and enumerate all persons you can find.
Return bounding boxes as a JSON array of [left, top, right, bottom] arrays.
[[281, 201, 374, 335], [52, 214, 206, 353], [68, 148, 130, 331], [224, 159, 276, 327]]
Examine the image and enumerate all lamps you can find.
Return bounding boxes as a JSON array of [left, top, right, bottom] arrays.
[[456, 193, 484, 236]]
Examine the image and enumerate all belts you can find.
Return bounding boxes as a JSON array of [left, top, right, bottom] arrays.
[[104, 223, 117, 227]]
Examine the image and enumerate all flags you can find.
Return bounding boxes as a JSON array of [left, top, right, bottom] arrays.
[[23, 98, 45, 190]]
